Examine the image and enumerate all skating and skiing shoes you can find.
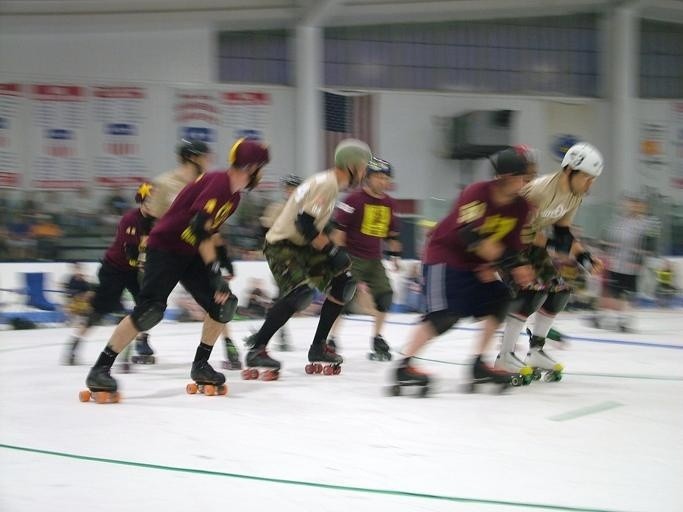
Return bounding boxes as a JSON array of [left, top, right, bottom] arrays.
[[79, 366, 119, 404], [131, 337, 155, 364], [467, 363, 510, 394], [368, 345, 391, 361], [390, 369, 431, 397], [187, 360, 227, 395], [242, 351, 281, 380], [495, 353, 534, 386], [524, 347, 563, 382], [305, 345, 344, 374], [61, 342, 76, 365], [222, 343, 240, 370], [113, 358, 128, 373]]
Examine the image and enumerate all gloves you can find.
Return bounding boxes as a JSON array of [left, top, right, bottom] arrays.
[[547, 277, 570, 294], [221, 261, 235, 281], [322, 242, 353, 276], [575, 250, 594, 274], [309, 261, 329, 281], [210, 274, 229, 291]]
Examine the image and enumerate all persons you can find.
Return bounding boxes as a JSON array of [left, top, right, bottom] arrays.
[[494, 142, 607, 377], [589, 186, 662, 334], [65, 180, 155, 372], [244, 138, 373, 371], [514, 145, 538, 187], [133, 137, 241, 363], [243, 172, 305, 352], [325, 155, 405, 353], [394, 147, 536, 385], [84, 134, 274, 393]]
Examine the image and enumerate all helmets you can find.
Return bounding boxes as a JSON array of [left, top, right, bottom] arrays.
[[334, 139, 373, 168], [560, 142, 604, 177], [175, 139, 216, 163], [230, 138, 273, 170], [363, 157, 393, 180], [280, 174, 302, 186], [497, 144, 537, 178]]
